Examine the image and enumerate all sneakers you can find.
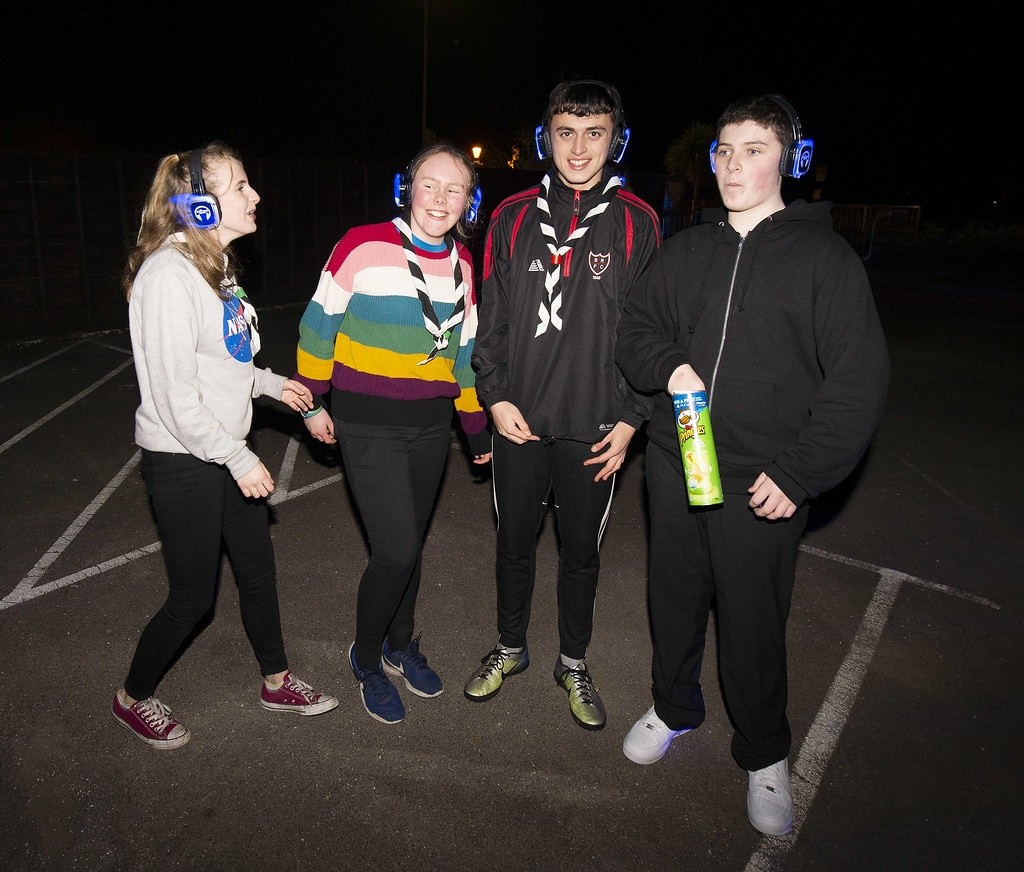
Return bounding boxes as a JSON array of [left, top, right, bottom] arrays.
[[260, 672, 339, 716], [746, 757, 793, 836], [111, 689, 192, 749], [553, 653, 607, 731], [348, 641, 405, 724], [463, 642, 529, 701], [381, 632, 444, 698], [622, 703, 691, 765]]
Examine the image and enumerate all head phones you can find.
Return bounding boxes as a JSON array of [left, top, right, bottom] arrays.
[[709, 95, 814, 179], [535, 80, 631, 164], [186, 149, 221, 230], [394, 144, 483, 227]]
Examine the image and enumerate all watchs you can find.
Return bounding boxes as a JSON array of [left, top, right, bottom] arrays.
[[301, 405, 322, 418]]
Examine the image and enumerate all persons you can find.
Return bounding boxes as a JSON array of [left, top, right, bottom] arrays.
[[110, 142, 340, 750], [463, 79, 664, 731], [614, 97, 890, 835], [293, 144, 493, 725]]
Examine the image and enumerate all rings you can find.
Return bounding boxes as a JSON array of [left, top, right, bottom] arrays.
[[253, 490, 258, 497]]
